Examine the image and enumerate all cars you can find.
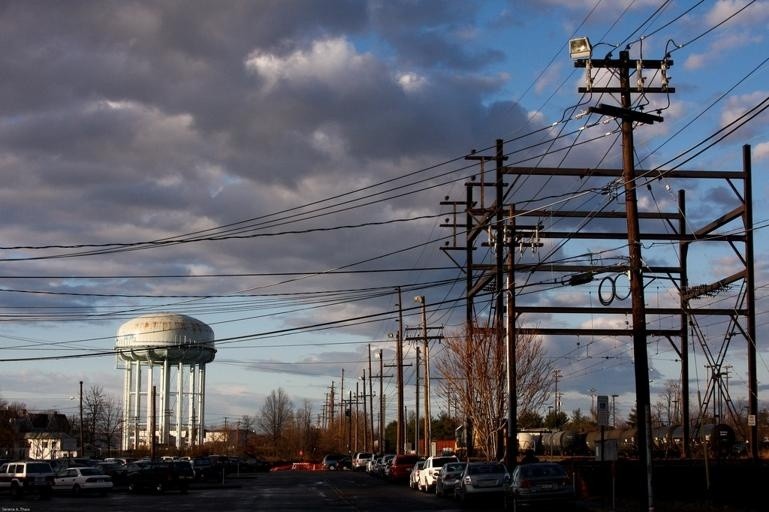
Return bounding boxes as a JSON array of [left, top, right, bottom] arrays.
[[453, 462, 511, 503], [503, 462, 572, 509], [1, 455, 269, 498], [433, 462, 466, 498], [335, 448, 461, 494]]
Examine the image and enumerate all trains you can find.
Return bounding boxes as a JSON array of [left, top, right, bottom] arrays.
[[538, 413, 745, 461]]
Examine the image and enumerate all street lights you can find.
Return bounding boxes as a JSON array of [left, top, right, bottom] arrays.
[[359, 369, 368, 451], [412, 295, 432, 460], [375, 349, 384, 454], [565, 36, 656, 509]]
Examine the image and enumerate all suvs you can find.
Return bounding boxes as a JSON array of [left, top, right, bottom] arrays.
[[323, 453, 349, 472]]
